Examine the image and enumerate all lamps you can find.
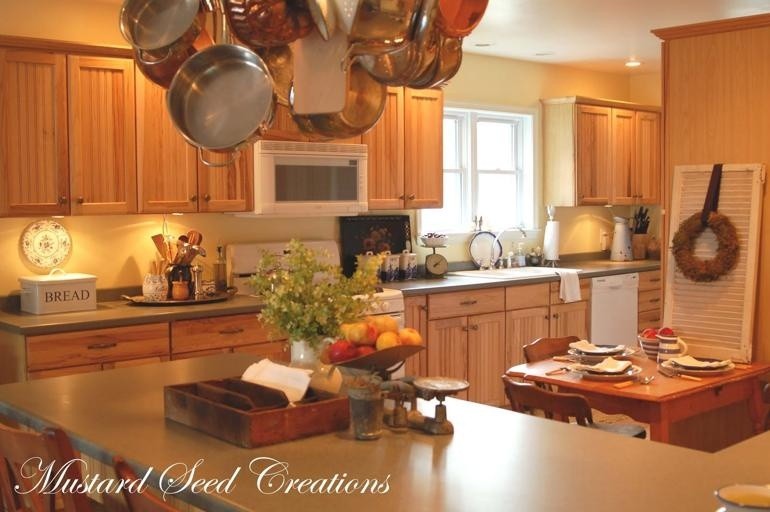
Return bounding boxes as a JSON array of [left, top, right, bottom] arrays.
[[626, 56, 641, 69]]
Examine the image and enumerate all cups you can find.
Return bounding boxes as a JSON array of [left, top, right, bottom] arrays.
[[346, 374, 384, 441], [657, 335, 687, 365]]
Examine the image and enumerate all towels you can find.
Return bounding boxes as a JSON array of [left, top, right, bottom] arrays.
[[554, 269, 583, 304], [670, 354, 731, 370], [570, 356, 631, 374], [569, 340, 625, 353]]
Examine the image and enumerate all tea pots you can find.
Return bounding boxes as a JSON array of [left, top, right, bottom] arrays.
[[610, 215, 633, 261]]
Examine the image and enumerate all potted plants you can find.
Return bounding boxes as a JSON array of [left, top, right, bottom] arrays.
[[246, 240, 390, 371]]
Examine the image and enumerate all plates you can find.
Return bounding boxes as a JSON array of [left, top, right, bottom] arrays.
[[567, 344, 635, 363], [20, 219, 73, 267], [414, 374, 469, 394], [661, 359, 736, 376], [326, 344, 429, 374], [468, 232, 503, 268], [420, 245, 447, 250], [120, 286, 240, 306], [581, 364, 642, 381]]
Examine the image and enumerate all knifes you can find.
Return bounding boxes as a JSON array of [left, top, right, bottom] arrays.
[[633, 207, 648, 259]]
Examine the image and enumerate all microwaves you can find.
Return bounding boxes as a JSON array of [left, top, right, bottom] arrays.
[[224, 140, 369, 219]]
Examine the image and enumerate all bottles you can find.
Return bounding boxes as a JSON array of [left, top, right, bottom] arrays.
[[212, 246, 228, 292], [518, 242, 526, 267]]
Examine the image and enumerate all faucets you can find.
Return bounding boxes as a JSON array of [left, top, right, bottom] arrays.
[[488, 227, 527, 271]]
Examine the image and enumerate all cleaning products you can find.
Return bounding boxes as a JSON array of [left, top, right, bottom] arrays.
[[516, 242, 525, 266]]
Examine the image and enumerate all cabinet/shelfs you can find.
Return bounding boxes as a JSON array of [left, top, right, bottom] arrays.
[[1, 51, 135, 215], [614, 109, 662, 206], [362, 86, 444, 210], [135, 61, 254, 211], [406, 295, 428, 380], [664, 163, 764, 364], [542, 97, 611, 208], [429, 286, 506, 407], [506, 279, 589, 407], [261, 89, 363, 143], [170, 312, 291, 363], [25, 321, 170, 380], [639, 268, 662, 332]]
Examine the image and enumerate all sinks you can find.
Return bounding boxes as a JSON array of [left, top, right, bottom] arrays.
[[447, 266, 582, 278]]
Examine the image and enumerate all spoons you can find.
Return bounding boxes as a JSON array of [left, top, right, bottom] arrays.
[[614, 375, 655, 388], [185, 243, 208, 266]]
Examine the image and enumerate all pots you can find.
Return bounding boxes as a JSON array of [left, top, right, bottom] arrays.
[[166, 42, 277, 169], [340, 0, 489, 88], [125, 44, 200, 91], [118, 0, 208, 64], [287, 58, 387, 142]]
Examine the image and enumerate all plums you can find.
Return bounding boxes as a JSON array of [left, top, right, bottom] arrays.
[[328, 340, 356, 361], [346, 322, 377, 347], [399, 327, 422, 346], [374, 315, 398, 335], [319, 344, 333, 366], [376, 332, 401, 350], [355, 345, 375, 357]]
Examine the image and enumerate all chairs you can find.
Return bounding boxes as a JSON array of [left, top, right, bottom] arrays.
[[523, 336, 647, 439], [112, 456, 184, 512], [0, 420, 92, 512], [501, 375, 593, 427]]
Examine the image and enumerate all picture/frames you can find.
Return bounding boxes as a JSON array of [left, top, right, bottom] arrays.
[[341, 214, 413, 280]]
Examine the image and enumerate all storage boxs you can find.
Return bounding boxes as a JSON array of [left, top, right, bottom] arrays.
[[166, 375, 350, 449], [19, 269, 96, 315]]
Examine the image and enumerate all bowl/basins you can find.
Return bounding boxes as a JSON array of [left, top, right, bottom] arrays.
[[637, 328, 657, 360], [420, 235, 449, 246]]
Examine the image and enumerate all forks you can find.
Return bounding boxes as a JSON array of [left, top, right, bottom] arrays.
[[658, 367, 702, 381]]
